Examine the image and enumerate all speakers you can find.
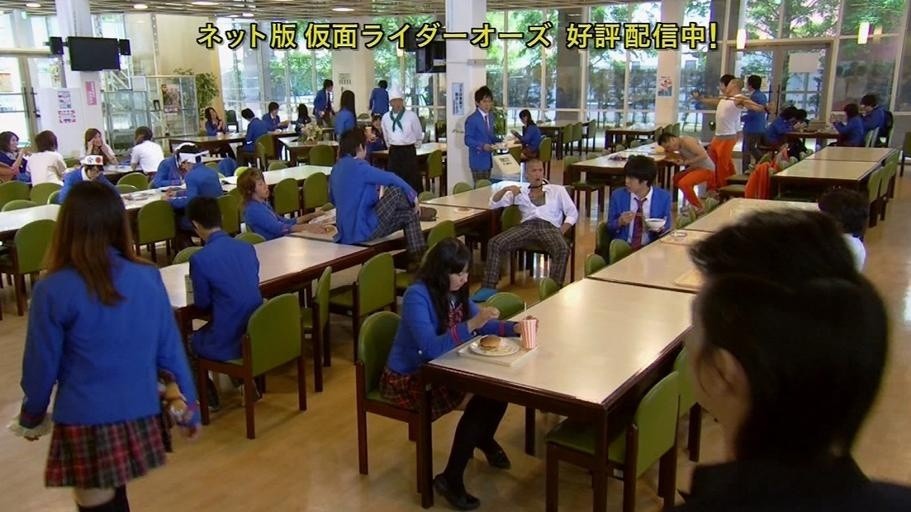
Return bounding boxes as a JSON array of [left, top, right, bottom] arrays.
[[49, 36, 64, 55], [119, 39, 131, 55]]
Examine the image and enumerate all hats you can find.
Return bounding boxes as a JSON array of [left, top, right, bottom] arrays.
[[389, 88, 403, 102]]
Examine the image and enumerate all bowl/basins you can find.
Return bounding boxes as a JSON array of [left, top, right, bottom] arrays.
[[644, 217, 666, 230]]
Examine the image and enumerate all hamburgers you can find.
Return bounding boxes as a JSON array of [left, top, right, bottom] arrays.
[[480, 335, 501, 351]]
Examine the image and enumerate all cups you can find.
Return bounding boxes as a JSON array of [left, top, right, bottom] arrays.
[[216, 132, 224, 140], [519, 319, 538, 349], [438, 137, 446, 150]]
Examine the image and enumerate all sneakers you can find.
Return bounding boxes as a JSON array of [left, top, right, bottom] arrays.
[[238, 379, 260, 406], [419, 207, 436, 220], [471, 287, 498, 301], [197, 398, 221, 411]]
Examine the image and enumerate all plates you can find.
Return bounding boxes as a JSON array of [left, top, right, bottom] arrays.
[[669, 230, 689, 241], [646, 148, 657, 155], [452, 206, 475, 215], [469, 334, 520, 356], [130, 192, 150, 203]]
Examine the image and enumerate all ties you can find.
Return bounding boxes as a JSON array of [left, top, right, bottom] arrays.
[[485, 116, 488, 127], [630, 200, 643, 251], [328, 93, 331, 112]]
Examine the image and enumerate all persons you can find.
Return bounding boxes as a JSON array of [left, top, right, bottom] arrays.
[[669, 211, 911, 511], [179, 196, 268, 414], [0, 74, 889, 305], [379, 236, 540, 510], [7, 179, 201, 512]]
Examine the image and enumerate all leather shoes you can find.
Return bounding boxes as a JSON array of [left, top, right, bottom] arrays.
[[433, 473, 480, 510], [477, 439, 511, 468]]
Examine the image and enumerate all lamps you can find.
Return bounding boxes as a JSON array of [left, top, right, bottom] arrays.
[[395, 35, 405, 60], [857, 21, 870, 46], [735, 27, 748, 52]]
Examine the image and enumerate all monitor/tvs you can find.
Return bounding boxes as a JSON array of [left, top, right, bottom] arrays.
[[66, 36, 121, 71], [415, 27, 447, 74]]
